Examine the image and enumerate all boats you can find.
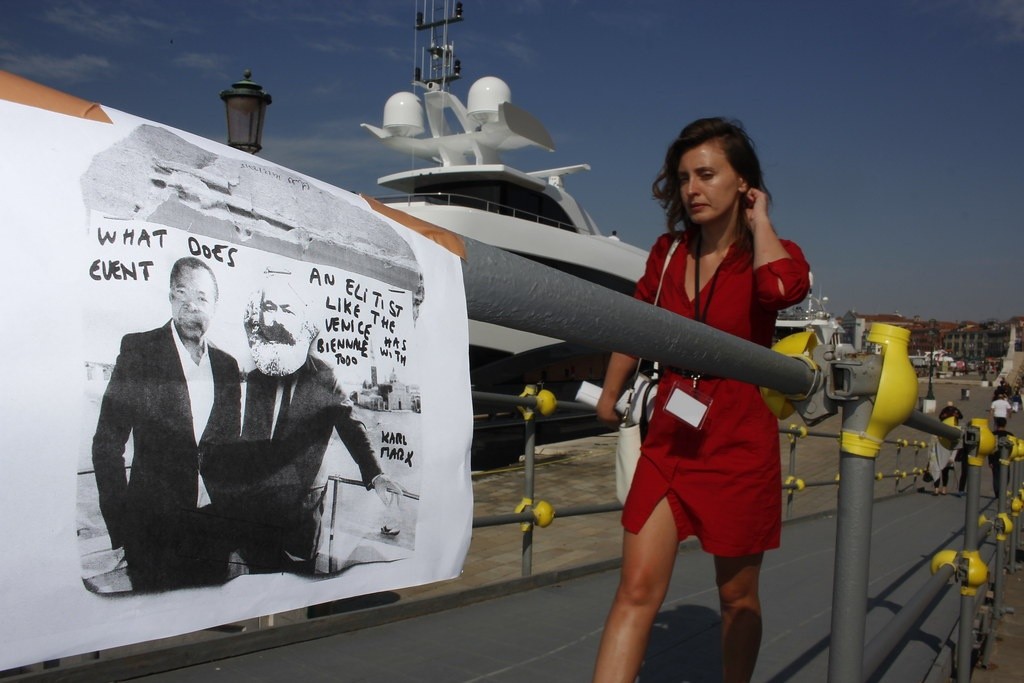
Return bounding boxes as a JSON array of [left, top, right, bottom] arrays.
[[360, 0, 845, 458]]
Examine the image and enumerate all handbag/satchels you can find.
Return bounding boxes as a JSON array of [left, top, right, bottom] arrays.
[[613, 384, 665, 510]]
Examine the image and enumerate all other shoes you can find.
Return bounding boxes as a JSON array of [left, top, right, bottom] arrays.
[[932, 487, 940, 495], [957, 491, 965, 497], [941, 486, 948, 495]]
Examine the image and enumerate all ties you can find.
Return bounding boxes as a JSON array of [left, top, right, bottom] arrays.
[[272, 373, 295, 451]]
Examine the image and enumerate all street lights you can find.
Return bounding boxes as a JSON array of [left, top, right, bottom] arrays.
[[925, 319, 935, 400]]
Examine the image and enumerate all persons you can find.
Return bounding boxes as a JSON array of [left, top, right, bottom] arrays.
[[90, 257, 408, 592], [595, 116, 811, 683], [929, 353, 1023, 496]]
